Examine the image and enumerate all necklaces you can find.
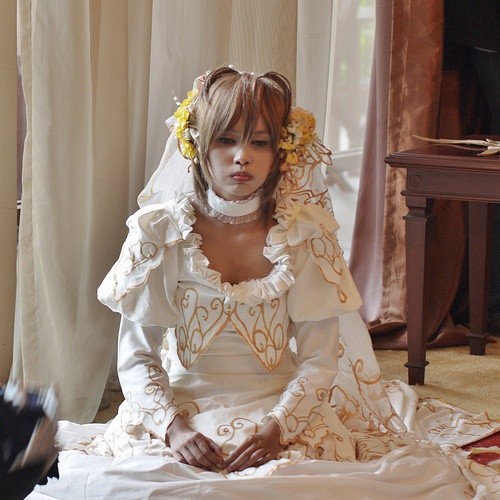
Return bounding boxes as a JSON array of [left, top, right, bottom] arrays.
[[203, 188, 268, 225]]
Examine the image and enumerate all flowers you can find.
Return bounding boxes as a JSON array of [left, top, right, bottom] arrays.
[[163, 84, 197, 163], [274, 105, 319, 179]]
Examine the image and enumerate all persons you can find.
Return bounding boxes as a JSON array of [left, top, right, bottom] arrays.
[[56, 68, 500, 500]]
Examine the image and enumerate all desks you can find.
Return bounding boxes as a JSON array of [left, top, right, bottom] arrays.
[[383, 141, 500, 386]]
